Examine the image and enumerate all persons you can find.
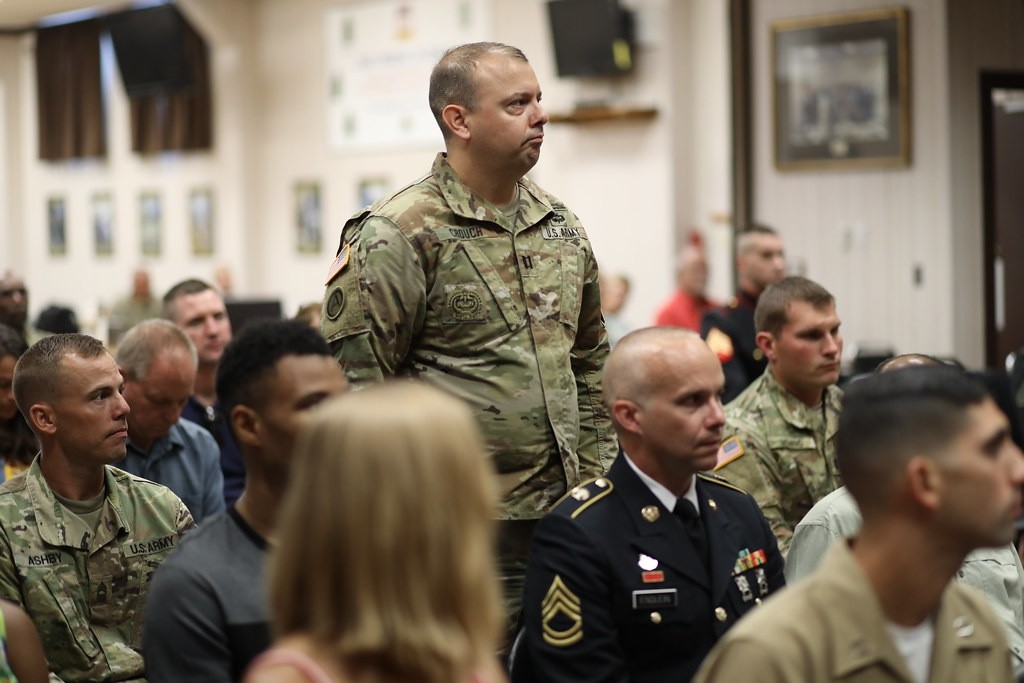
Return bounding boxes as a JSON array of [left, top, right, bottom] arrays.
[[321, 42, 620, 669], [0, 227, 1024, 683]]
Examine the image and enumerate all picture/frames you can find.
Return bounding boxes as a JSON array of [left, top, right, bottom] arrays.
[[188, 186, 216, 254], [136, 190, 167, 258], [770, 6, 918, 171], [292, 178, 323, 252], [48, 198, 69, 254], [93, 195, 120, 255]]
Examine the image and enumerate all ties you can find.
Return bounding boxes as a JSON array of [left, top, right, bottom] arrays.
[[671, 498, 713, 579]]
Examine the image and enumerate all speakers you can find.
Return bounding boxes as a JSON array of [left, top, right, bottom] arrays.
[[548, 0, 631, 78]]
